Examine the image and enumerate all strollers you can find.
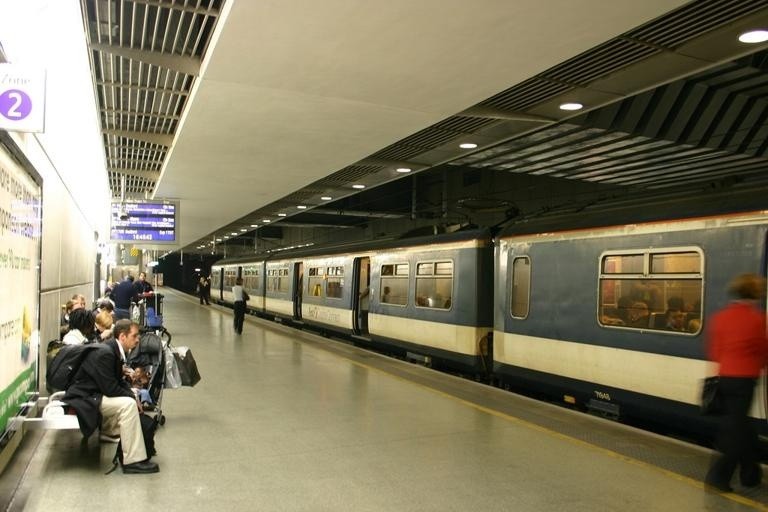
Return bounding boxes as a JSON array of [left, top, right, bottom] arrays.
[[121, 324, 171, 429]]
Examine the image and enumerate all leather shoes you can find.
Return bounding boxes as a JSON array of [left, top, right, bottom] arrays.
[[123, 461, 160, 474]]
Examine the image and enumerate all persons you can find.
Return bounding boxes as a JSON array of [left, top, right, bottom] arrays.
[[197, 267, 210, 305], [45, 272, 159, 474], [382, 286, 390, 303], [231, 277, 250, 334], [603, 279, 701, 333], [703, 273, 767, 497]]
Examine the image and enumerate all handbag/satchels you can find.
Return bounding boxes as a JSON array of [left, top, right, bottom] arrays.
[[163, 347, 181, 390], [696, 375, 721, 430], [174, 345, 201, 387], [242, 290, 249, 302]]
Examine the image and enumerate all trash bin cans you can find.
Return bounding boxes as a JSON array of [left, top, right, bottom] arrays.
[[137, 290, 164, 328]]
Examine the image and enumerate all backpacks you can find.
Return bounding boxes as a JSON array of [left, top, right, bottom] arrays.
[[111, 414, 156, 467], [47, 343, 115, 389]]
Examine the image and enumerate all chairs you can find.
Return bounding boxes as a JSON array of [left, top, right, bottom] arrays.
[[649, 312, 700, 332], [603, 303, 619, 319]]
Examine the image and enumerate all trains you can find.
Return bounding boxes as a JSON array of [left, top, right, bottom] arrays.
[[208, 204, 768, 463]]
